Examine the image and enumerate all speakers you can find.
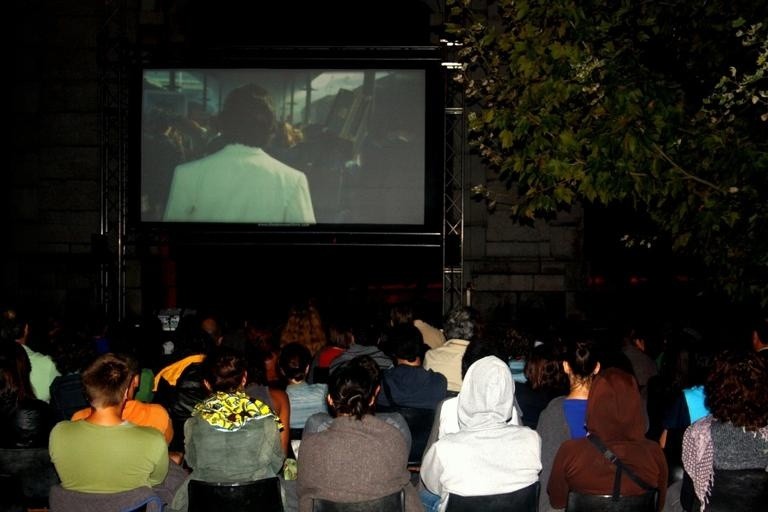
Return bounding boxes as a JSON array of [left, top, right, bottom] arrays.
[[466, 290, 566, 340]]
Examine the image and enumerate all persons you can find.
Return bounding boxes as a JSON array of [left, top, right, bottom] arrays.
[[2, 309, 478, 512], [144, 86, 413, 223], [418, 310, 767, 512]]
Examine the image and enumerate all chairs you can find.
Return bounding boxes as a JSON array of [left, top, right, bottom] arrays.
[[567, 487, 659, 512], [691, 468, 767, 511], [378, 404, 435, 462], [186, 476, 287, 512], [312, 488, 406, 511], [0, 446, 57, 509], [444, 481, 541, 512]]
